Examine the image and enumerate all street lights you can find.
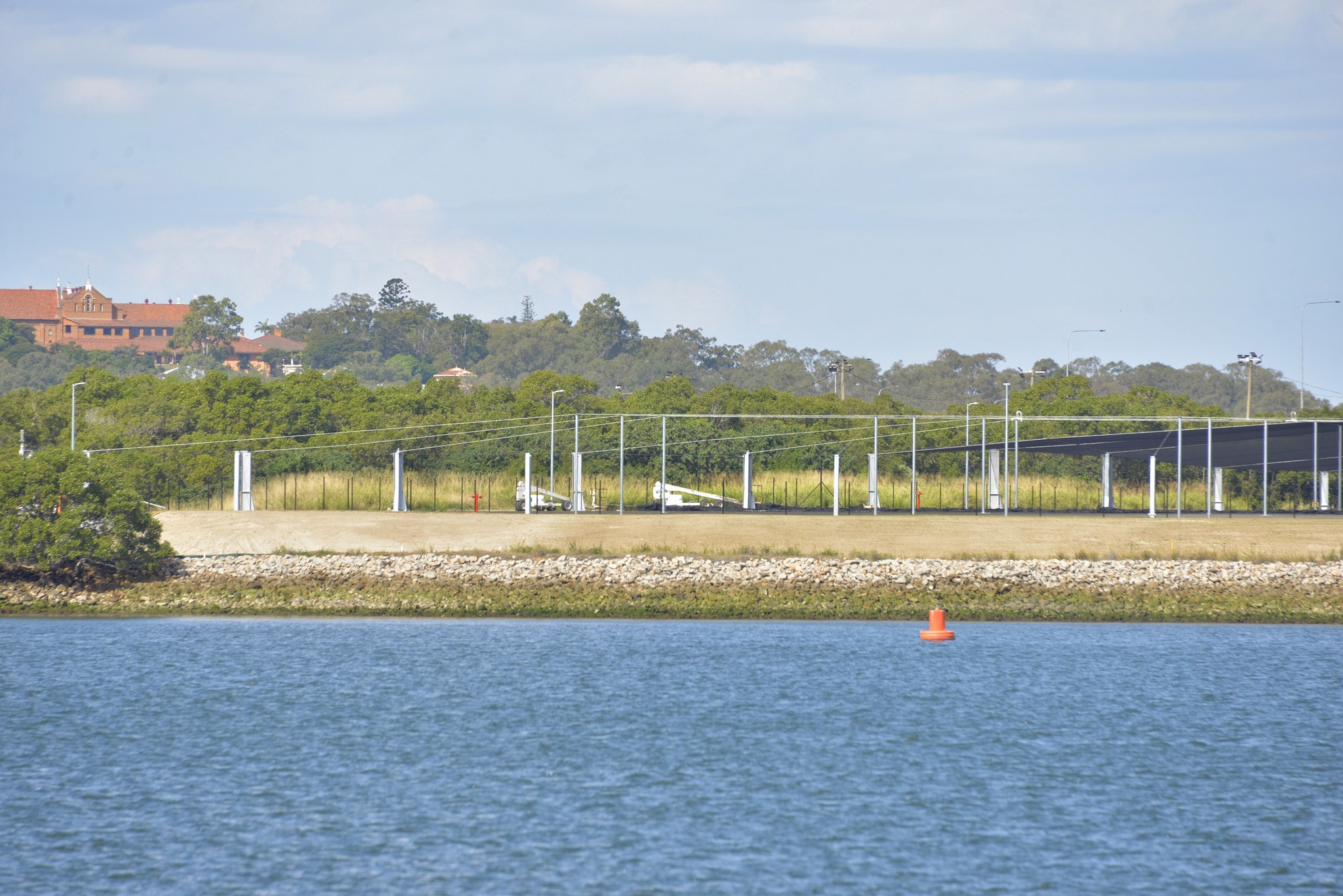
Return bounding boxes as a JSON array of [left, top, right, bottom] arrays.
[[72, 381, 88, 451], [965, 402, 978, 508], [829, 359, 854, 400], [1067, 329, 1106, 377], [856, 382, 900, 397], [1003, 382, 1011, 514], [1018, 367, 1048, 387], [1238, 352, 1263, 419], [1299, 300, 1342, 409], [550, 389, 565, 501]]
